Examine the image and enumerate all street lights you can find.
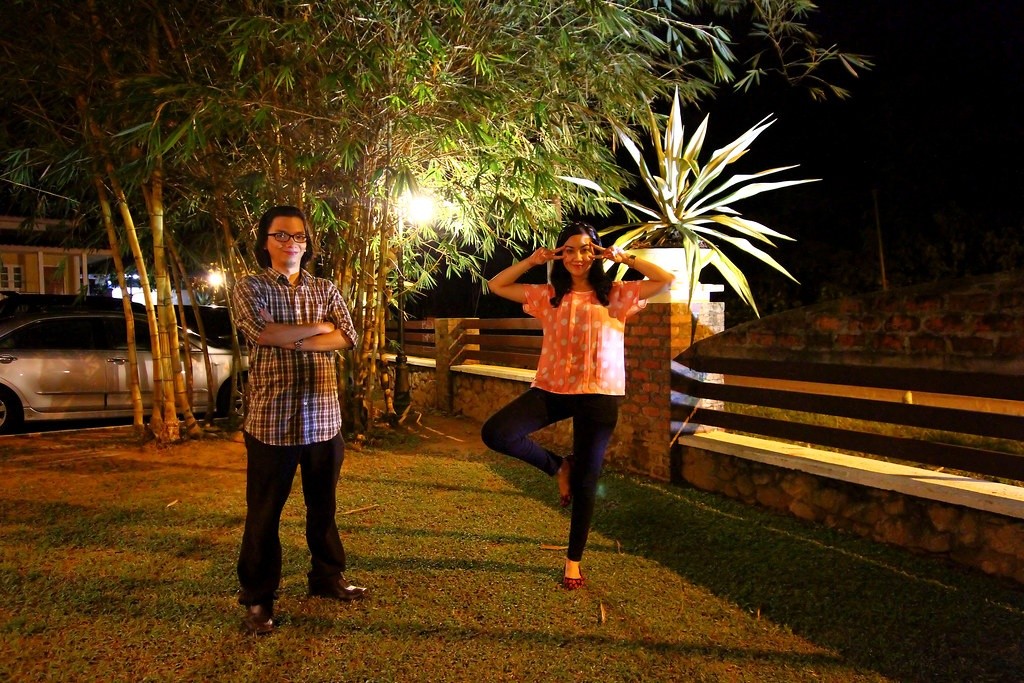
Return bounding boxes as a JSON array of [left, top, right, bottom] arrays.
[[394, 192, 434, 415]]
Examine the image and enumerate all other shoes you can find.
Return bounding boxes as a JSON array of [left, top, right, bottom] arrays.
[[559, 455, 573, 508], [563, 557, 584, 591]]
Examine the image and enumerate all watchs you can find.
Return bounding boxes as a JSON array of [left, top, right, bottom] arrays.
[[294, 339, 303, 351]]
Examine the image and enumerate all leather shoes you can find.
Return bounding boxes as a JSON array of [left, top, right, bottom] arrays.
[[245, 600, 274, 633], [307, 571, 367, 598]]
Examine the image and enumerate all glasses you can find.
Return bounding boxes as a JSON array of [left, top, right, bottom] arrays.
[[267, 232, 309, 243]]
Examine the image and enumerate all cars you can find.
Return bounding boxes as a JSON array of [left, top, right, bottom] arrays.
[[0, 290, 250, 434]]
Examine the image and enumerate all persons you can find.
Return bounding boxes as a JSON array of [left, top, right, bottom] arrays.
[[233, 206, 369, 632], [480, 223, 676, 590]]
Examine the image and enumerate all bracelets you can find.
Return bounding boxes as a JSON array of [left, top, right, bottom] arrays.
[[628, 255, 636, 269]]
